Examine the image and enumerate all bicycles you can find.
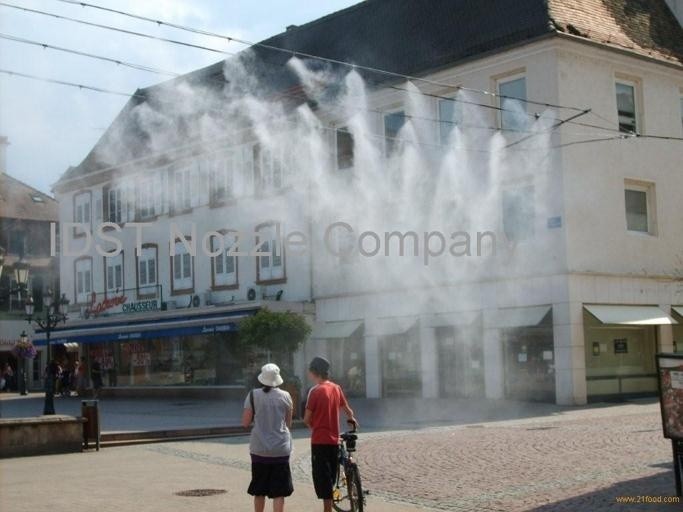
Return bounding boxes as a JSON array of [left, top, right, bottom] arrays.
[[331, 420, 370, 511]]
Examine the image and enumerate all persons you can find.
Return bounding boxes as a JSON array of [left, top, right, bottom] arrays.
[[240, 363, 295, 511], [44, 351, 103, 399], [1, 364, 13, 392], [0, 362, 8, 390], [107, 352, 118, 387], [302, 356, 360, 512]]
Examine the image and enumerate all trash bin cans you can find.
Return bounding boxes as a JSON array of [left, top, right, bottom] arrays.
[[81, 399, 101, 452]]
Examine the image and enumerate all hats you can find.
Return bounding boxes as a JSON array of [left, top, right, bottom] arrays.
[[258, 363, 284, 387], [311, 355, 329, 373]]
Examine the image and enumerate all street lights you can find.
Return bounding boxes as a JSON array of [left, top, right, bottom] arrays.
[[0, 245, 28, 300], [22, 289, 70, 414]]
